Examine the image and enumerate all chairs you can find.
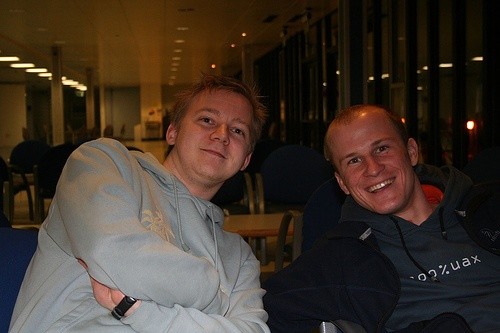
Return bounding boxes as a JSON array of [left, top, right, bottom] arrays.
[[0, 139, 98, 333], [275, 177, 369, 333], [213, 171, 267, 266]]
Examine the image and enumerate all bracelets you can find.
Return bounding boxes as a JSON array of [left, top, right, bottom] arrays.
[[111, 296, 137, 320]]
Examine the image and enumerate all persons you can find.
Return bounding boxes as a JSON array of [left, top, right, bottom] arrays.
[[261, 104, 499, 332], [8, 76, 271, 333]]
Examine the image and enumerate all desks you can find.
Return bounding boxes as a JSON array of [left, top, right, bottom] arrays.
[[222, 211, 294, 239]]
